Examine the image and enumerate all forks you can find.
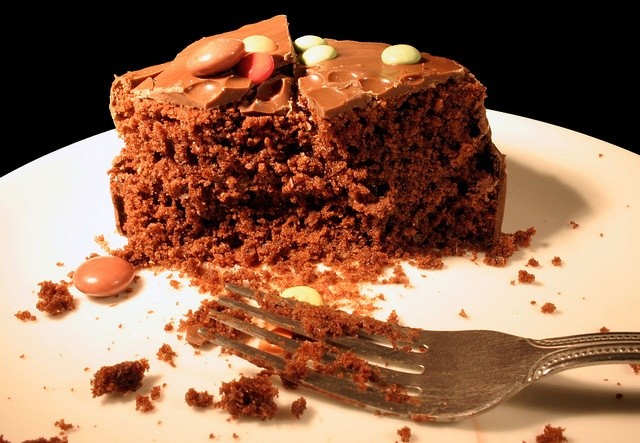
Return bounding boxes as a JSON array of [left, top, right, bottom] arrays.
[[197, 283, 640, 422]]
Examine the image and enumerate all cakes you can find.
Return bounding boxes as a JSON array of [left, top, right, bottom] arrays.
[[109, 14, 508, 275]]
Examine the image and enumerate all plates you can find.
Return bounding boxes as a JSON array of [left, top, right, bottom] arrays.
[[0, 107, 640, 443]]
